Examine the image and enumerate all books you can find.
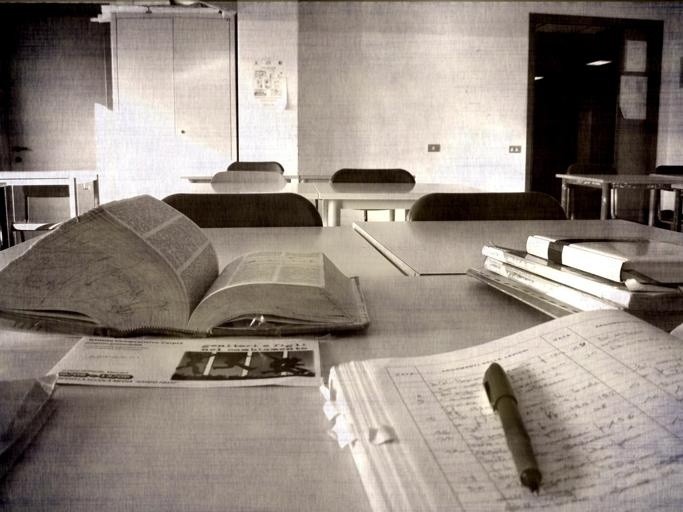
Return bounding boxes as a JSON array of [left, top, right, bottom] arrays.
[[0, 378, 57, 479], [0, 195, 371, 339], [329, 232, 683, 512]]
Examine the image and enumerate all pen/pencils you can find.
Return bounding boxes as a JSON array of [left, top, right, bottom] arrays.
[[482, 363, 542, 497]]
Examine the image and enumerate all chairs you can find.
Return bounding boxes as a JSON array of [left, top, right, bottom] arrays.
[[212, 171, 284, 183], [409, 193, 566, 220], [329, 168, 415, 222], [162, 192, 323, 227], [228, 161, 283, 175]]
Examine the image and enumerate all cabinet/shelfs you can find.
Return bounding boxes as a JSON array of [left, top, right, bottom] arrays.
[[110, 11, 239, 205]]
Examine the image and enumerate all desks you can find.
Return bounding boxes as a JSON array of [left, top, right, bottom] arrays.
[[187, 184, 319, 213], [0, 273, 683, 511], [0, 227, 408, 280], [556, 174, 683, 233], [0, 170, 99, 251], [180, 176, 333, 183], [314, 183, 484, 227], [352, 219, 683, 278]]
